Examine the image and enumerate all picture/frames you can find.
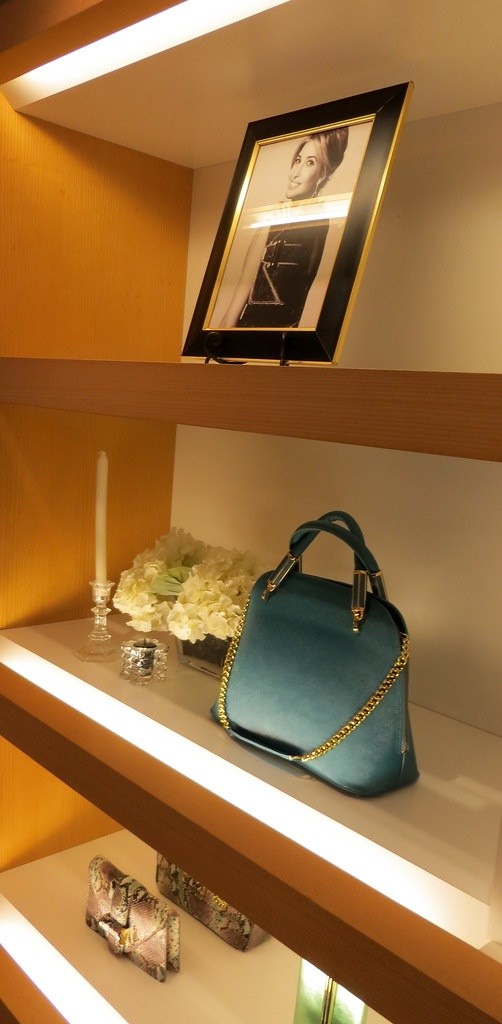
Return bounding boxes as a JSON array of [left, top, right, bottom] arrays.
[[182, 83, 415, 364]]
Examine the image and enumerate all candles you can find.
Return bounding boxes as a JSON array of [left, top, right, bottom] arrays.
[[94, 448, 109, 584], [132, 637, 156, 679]]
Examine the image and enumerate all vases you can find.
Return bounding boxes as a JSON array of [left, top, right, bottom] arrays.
[[173, 635, 234, 679]]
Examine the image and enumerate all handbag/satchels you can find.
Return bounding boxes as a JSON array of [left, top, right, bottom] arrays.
[[154, 852, 269, 954], [84, 856, 181, 983], [210, 507, 420, 798]]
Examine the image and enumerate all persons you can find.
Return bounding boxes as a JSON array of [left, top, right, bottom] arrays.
[[218, 126, 350, 329]]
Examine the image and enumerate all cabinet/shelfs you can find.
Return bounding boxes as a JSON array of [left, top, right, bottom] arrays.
[[0, 0, 502, 1023]]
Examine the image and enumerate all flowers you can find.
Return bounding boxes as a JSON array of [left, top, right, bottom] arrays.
[[113, 525, 263, 645]]
